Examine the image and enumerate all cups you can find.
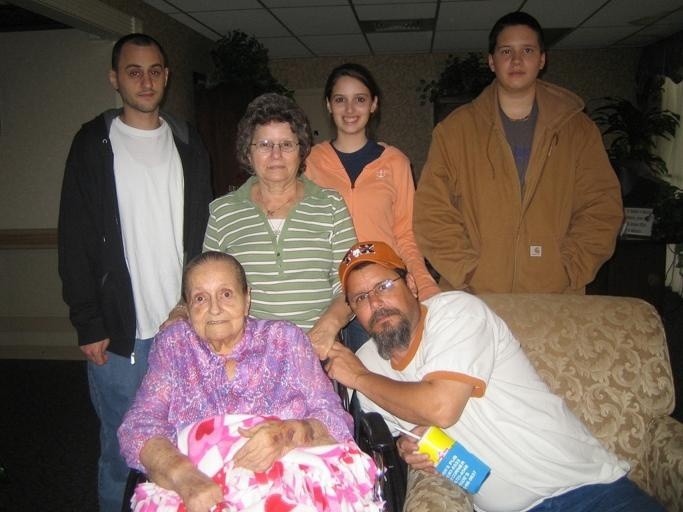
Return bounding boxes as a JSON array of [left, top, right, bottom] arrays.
[[412, 424, 492, 496]]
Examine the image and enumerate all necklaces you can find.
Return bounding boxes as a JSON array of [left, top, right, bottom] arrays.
[[255, 185, 295, 237]]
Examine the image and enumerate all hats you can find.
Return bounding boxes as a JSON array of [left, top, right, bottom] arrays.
[[339, 241, 407, 294]]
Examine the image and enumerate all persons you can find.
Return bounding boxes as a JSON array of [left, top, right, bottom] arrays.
[[309, 239, 668, 512], [116, 250, 357, 512], [197, 91, 359, 345], [301, 60, 445, 354], [55, 30, 214, 511], [414, 8, 628, 296]]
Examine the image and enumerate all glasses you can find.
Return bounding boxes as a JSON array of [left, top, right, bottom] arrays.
[[251, 138, 299, 152], [352, 277, 400, 303]]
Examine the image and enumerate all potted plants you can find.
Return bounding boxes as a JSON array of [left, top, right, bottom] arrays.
[[585, 70, 680, 178]]
